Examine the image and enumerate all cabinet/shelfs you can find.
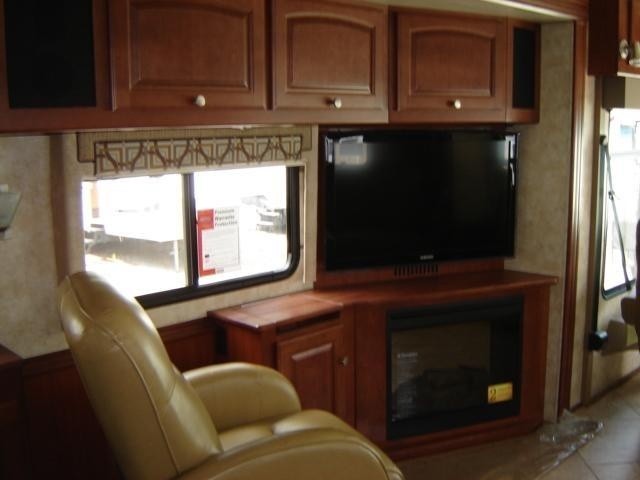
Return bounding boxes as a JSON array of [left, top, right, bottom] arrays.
[[206, 270, 559, 463], [511, 20, 539, 123], [273, 324, 350, 428], [269, 3, 384, 111], [303, 276, 554, 457], [110, 1, 267, 114], [587, 0, 640, 78], [391, 15, 509, 122]]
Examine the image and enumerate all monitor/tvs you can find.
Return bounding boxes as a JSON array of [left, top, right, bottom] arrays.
[[317, 122, 520, 272]]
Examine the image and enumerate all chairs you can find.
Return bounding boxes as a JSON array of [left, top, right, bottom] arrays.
[[55, 271, 404, 480]]
